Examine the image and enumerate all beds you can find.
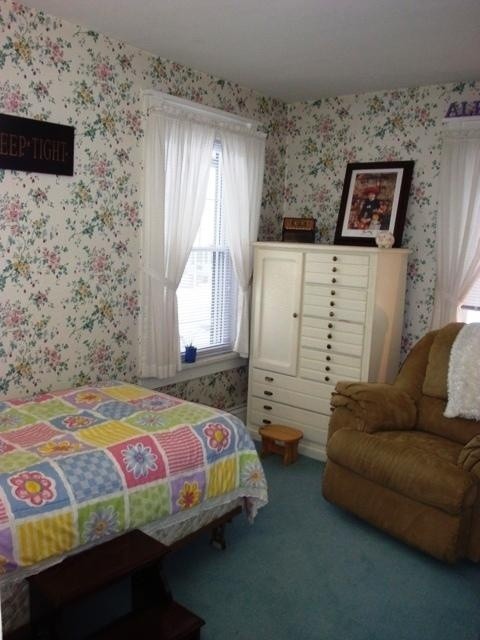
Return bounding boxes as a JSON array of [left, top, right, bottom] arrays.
[[0, 379, 269, 636]]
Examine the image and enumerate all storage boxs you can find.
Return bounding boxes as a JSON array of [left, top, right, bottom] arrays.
[[281, 217, 316, 242]]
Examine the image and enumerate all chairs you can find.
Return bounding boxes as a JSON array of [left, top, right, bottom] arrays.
[[320, 322, 479, 566]]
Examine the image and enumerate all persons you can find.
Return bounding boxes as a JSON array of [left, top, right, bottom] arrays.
[[368, 208, 382, 230], [353, 187, 385, 228]]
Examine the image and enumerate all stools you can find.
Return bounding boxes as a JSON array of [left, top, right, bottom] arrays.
[[259, 424, 304, 467]]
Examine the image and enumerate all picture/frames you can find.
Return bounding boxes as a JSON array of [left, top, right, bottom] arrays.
[[330, 159, 415, 248]]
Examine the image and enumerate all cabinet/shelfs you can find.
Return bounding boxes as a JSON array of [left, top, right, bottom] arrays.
[[25, 529, 207, 639], [246, 243, 411, 463]]
